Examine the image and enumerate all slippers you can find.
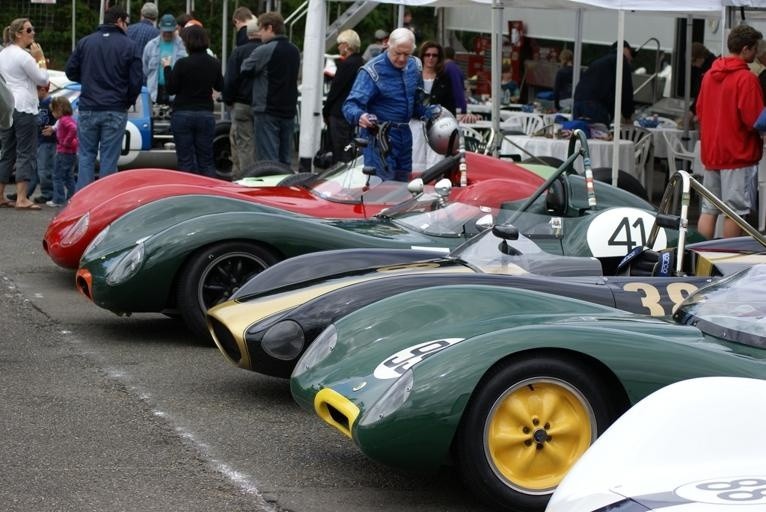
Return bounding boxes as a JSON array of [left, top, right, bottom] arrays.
[[15, 202, 42, 211], [0, 199, 16, 208]]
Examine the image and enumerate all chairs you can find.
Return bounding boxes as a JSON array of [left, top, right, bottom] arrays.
[[454, 103, 696, 189], [545, 178, 567, 215], [623, 248, 660, 276]]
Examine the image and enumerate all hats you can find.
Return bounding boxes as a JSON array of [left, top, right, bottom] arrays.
[[611, 40, 637, 53], [374, 29, 390, 40], [141, 2, 159, 19]]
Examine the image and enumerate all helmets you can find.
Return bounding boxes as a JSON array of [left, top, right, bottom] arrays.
[[158, 13, 177, 33], [424, 108, 460, 155]]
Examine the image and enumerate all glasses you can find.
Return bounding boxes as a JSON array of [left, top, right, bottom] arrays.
[[424, 53, 439, 58], [18, 26, 34, 34]]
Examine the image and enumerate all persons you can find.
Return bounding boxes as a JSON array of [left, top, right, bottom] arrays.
[[224, 6, 299, 176], [0, 18, 77, 207], [554, 49, 583, 111], [688, 24, 766, 238], [66, 7, 143, 193], [573, 39, 635, 125], [322, 8, 464, 182], [127, 4, 224, 178]]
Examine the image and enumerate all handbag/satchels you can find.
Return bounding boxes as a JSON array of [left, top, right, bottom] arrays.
[[314, 146, 335, 169]]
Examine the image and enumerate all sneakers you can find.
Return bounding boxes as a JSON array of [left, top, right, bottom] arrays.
[[33, 196, 53, 203], [6, 192, 30, 201], [45, 199, 66, 208]]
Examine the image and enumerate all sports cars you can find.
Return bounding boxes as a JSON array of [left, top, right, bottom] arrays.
[[9, 83, 277, 179], [43, 151, 764, 510]]
